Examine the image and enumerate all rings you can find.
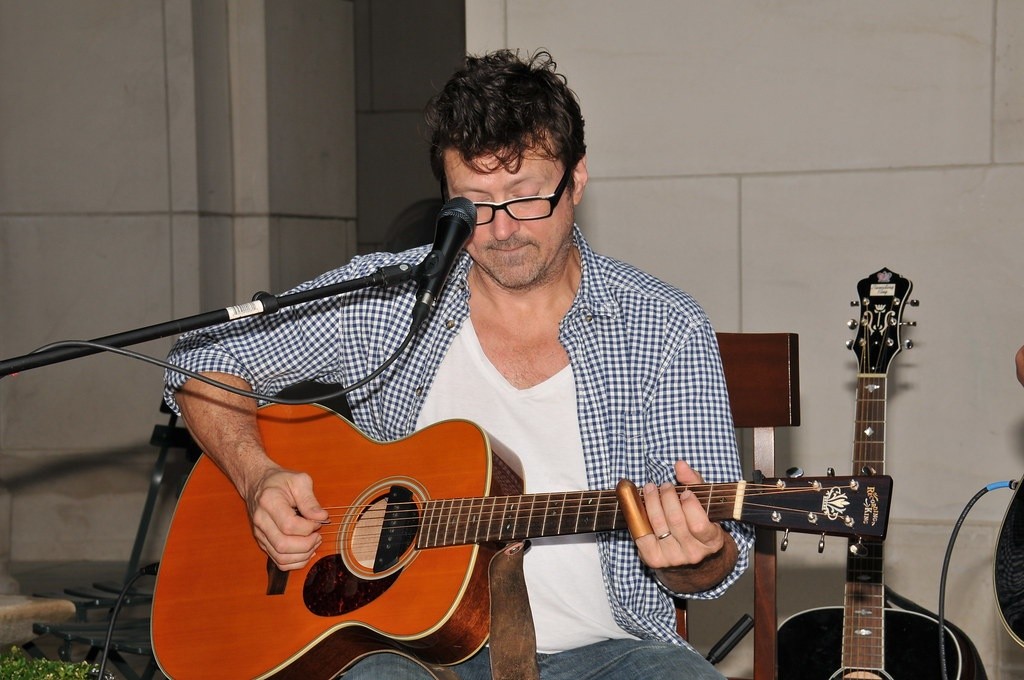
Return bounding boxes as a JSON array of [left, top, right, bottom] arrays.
[[658, 531, 673, 541]]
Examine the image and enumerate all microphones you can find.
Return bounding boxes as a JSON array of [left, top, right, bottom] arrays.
[[409, 196, 478, 333]]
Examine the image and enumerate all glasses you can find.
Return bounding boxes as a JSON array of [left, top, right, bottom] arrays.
[[437, 159, 573, 228]]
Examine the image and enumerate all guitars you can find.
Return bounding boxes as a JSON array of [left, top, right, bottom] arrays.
[[992, 472, 1024, 650], [149, 398, 894, 680], [773, 265, 995, 680]]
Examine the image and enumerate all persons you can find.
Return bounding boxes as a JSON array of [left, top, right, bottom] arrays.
[[162, 43, 759, 679]]
